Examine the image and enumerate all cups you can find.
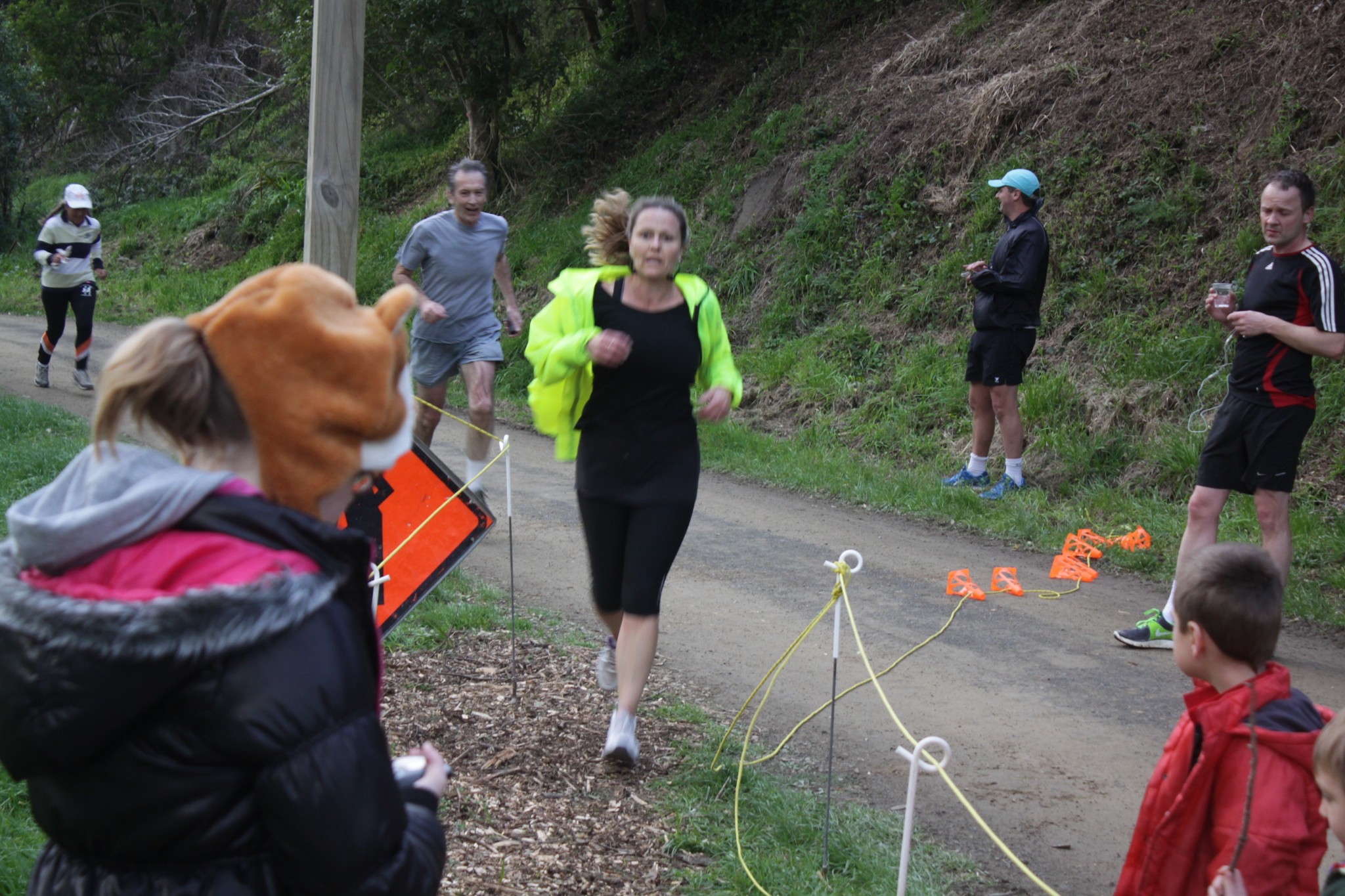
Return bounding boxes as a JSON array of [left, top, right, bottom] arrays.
[[1212, 283, 1232, 307]]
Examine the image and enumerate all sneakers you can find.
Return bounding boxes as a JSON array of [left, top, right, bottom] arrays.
[[596, 634, 619, 691], [72, 367, 94, 390], [34, 362, 50, 388], [1113, 609, 1176, 650], [474, 491, 490, 516], [601, 709, 641, 762], [977, 473, 1027, 500], [941, 466, 991, 490]]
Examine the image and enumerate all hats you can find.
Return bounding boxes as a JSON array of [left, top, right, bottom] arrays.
[[180, 262, 423, 520], [987, 169, 1042, 200], [64, 184, 94, 209]]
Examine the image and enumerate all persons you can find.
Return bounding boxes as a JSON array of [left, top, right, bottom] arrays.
[[1115, 538, 1340, 896], [1114, 167, 1345, 648], [521, 187, 746, 766], [1, 261, 458, 895], [391, 154, 521, 509], [1204, 704, 1343, 896], [940, 169, 1049, 499], [29, 183, 111, 394]]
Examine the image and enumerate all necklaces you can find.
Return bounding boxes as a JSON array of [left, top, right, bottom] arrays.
[[629, 284, 671, 309]]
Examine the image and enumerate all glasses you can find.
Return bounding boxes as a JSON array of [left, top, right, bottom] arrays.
[[347, 464, 386, 506]]
